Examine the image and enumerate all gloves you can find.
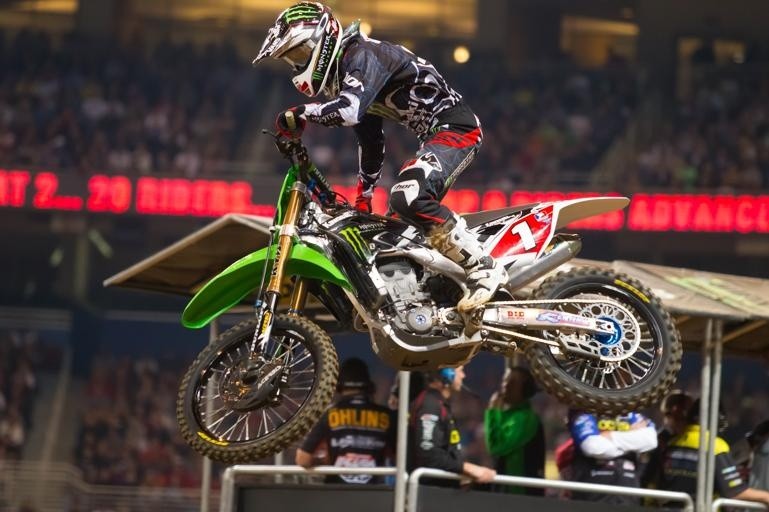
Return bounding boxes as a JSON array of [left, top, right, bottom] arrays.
[[276, 102, 320, 139], [354, 179, 373, 214]]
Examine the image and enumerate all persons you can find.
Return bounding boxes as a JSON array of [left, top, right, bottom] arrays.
[[253, 1, 512, 310]]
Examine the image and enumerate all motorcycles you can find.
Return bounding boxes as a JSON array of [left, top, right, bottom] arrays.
[[177, 120, 685, 466]]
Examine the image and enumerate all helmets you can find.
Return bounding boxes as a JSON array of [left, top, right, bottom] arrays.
[[252, 1, 343, 97], [335, 357, 373, 388]]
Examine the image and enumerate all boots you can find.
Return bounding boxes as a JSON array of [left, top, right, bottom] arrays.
[[425, 209, 509, 311]]
